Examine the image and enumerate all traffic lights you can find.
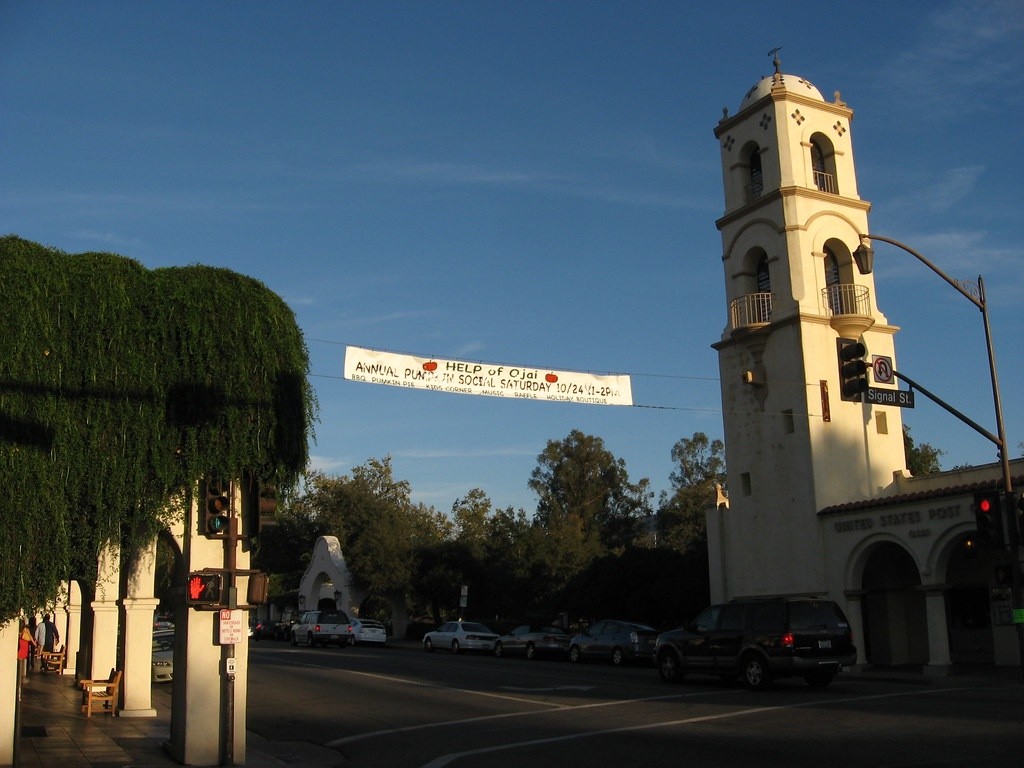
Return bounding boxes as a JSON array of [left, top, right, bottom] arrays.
[[837, 338, 870, 404], [972, 489, 1011, 553], [993, 564, 1011, 585], [257, 468, 281, 532], [187, 575, 223, 607], [205, 472, 228, 537]]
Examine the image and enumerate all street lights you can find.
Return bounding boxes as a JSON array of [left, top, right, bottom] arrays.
[[853, 232, 1023, 662], [960, 537, 976, 561]]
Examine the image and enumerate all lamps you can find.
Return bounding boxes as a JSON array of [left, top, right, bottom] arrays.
[[334, 589, 342, 606], [300, 594, 305, 609]]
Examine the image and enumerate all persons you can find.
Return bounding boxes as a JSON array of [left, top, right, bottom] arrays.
[[18, 627, 37, 683], [34, 613, 59, 669], [27, 617, 39, 669]]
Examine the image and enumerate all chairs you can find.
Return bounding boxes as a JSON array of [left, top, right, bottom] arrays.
[[80, 668, 122, 718], [42, 645, 66, 675]]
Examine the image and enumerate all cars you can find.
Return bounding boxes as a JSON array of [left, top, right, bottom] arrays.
[[347, 617, 387, 646], [495, 624, 576, 660], [152, 614, 174, 683], [567, 619, 662, 665], [249, 618, 271, 640], [421, 621, 500, 654]]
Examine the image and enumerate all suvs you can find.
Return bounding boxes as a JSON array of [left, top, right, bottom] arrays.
[[289, 610, 355, 646], [272, 609, 313, 641], [654, 595, 853, 690]]
